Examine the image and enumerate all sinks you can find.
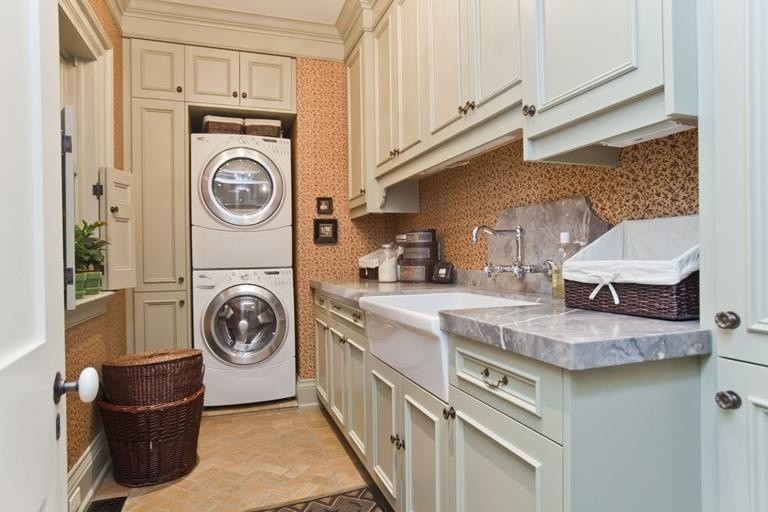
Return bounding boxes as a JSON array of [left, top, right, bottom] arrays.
[[357, 285, 546, 403]]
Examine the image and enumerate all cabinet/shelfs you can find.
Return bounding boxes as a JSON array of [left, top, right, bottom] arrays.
[[185, 45, 297, 110], [131, 97, 187, 293], [524, 2, 664, 154], [131, 38, 185, 101], [374, 0, 421, 165], [366, 352, 445, 512], [446, 331, 566, 511], [429, 0, 524, 142], [310, 288, 365, 334], [703, 6, 763, 502], [133, 290, 188, 354], [314, 313, 365, 467], [346, 20, 370, 201]]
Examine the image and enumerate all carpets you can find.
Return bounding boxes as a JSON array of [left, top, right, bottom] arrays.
[[243, 485, 389, 512]]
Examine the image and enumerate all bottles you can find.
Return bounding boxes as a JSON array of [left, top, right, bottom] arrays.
[[551, 232, 578, 298], [377, 243, 397, 282]]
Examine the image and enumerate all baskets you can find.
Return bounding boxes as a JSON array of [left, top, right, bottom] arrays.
[[101, 349, 206, 407], [97, 384, 207, 489]]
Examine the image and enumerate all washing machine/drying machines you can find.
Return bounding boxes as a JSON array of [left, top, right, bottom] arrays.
[[192, 269, 297, 407], [191, 148, 293, 269]]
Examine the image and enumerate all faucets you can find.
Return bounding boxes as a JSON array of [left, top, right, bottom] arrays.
[[471, 224, 552, 281]]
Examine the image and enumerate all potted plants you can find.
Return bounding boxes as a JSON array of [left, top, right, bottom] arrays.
[[75, 220, 111, 300]]
